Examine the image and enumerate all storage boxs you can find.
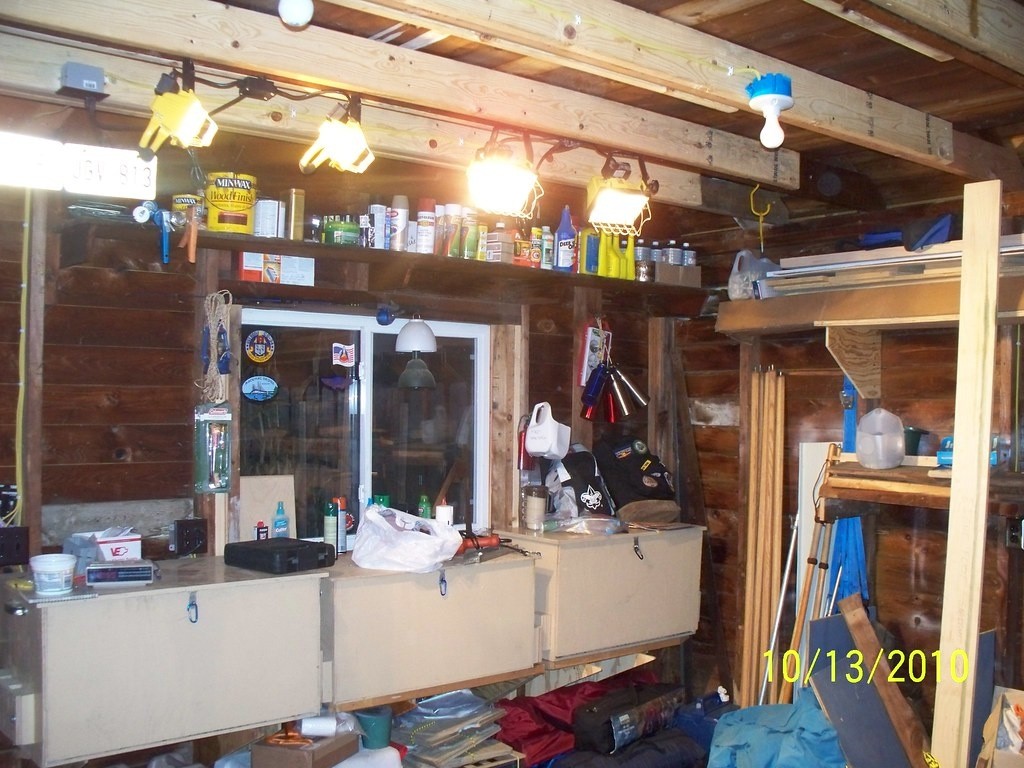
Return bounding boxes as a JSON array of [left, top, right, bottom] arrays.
[[635, 260, 702, 290], [250, 728, 359, 768], [990, 685, 1024, 768]]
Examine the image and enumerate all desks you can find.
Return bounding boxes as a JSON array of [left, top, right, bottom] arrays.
[[0, 524, 707, 768]]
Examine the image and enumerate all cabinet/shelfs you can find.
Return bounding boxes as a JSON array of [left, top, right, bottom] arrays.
[[713, 276, 1024, 514]]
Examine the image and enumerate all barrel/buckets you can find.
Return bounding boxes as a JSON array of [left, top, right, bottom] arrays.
[[171, 172, 286, 238]]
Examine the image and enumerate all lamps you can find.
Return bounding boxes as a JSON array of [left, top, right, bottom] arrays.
[[298, 102, 375, 175], [140, 74, 220, 151], [395, 313, 438, 389], [466, 145, 544, 218], [587, 174, 651, 235]]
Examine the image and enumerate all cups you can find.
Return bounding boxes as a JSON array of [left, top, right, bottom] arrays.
[[903, 426, 930, 455], [353, 705, 393, 749], [521, 485, 553, 530]]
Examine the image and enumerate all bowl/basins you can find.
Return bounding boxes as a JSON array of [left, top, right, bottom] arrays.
[[29, 553, 77, 595]]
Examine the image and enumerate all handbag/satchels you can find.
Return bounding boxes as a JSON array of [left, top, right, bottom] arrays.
[[387, 649, 849, 768], [350, 503, 463, 575], [562, 444, 616, 520], [596, 432, 685, 523]]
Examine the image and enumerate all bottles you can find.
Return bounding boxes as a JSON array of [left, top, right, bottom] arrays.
[[518, 420, 536, 471], [323, 494, 454, 560], [320, 192, 697, 283], [580, 363, 650, 425], [271, 501, 290, 538], [253, 522, 268, 540]]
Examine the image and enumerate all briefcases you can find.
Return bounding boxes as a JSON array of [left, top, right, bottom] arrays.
[[224, 536, 335, 577]]
